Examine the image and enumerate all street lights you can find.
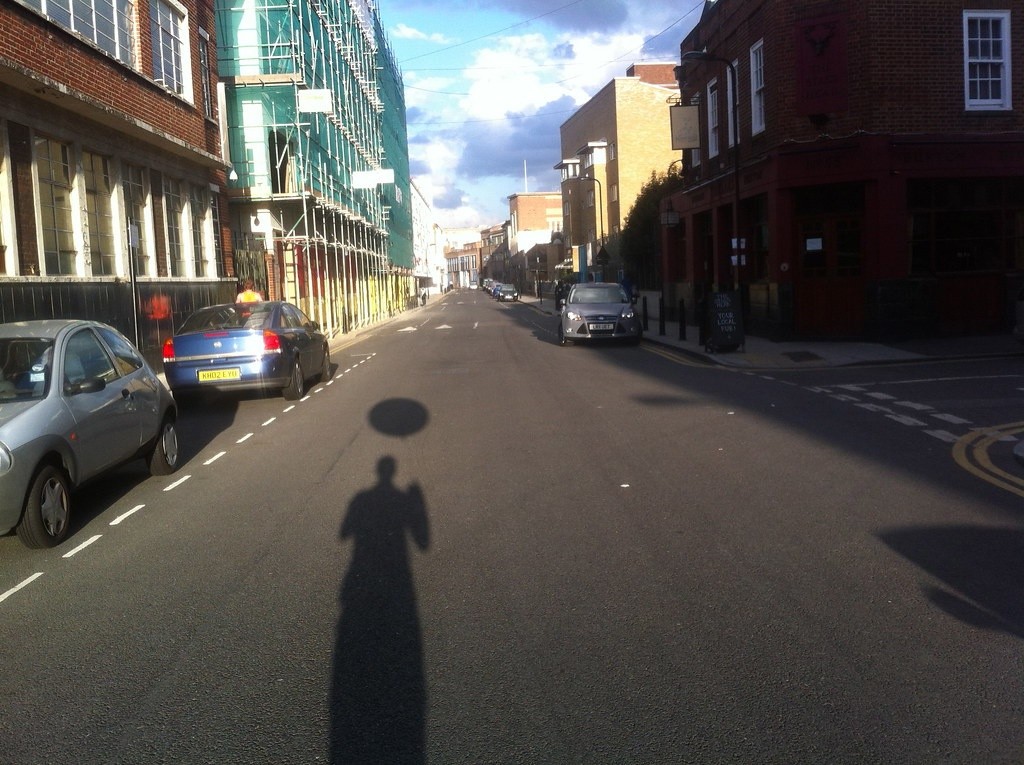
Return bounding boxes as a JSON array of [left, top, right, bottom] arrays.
[[577, 176, 606, 283]]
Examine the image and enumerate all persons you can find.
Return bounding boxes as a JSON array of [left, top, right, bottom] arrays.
[[236, 279, 263, 326]]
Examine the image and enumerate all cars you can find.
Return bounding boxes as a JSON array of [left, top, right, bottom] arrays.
[[559, 283, 643, 348], [1, 321, 183, 549], [162, 299, 331, 412], [499, 284, 519, 302], [481, 278, 502, 299]]
